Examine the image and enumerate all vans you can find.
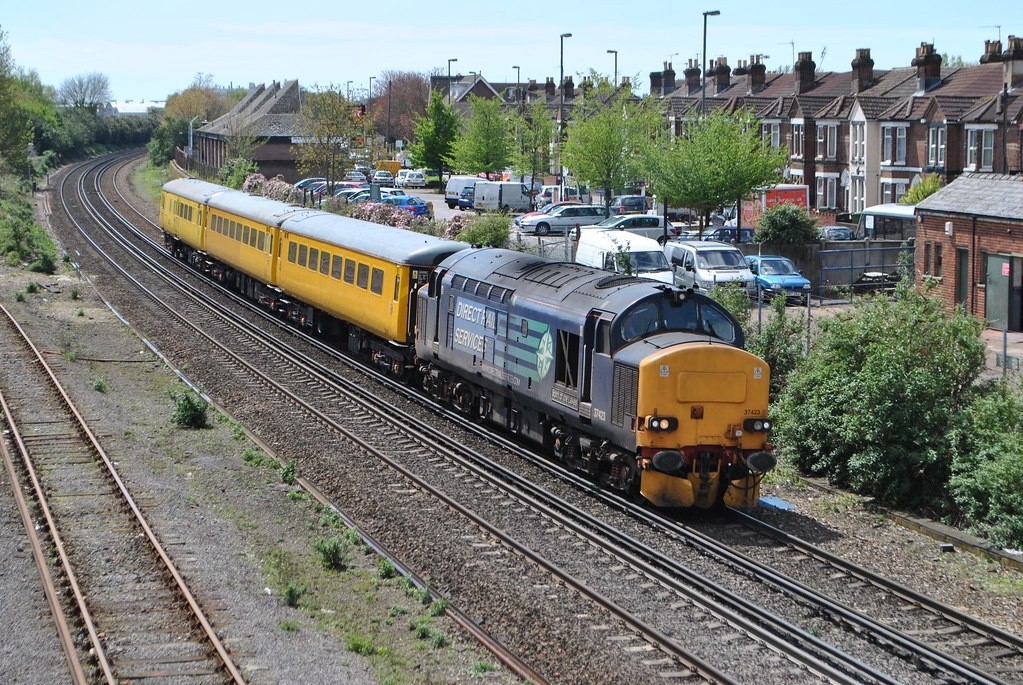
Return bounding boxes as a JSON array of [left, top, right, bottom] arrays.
[[576, 229, 675, 286], [662, 239, 756, 297], [473, 181, 538, 214], [445, 176, 490, 209]]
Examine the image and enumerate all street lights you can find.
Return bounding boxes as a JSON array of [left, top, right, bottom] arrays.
[[347, 33, 618, 180], [702, 11, 720, 122]]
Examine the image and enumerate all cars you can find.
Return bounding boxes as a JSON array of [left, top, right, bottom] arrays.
[[423, 168, 451, 185], [402, 172, 425, 189], [294, 178, 433, 221], [524, 182, 582, 208], [569, 214, 677, 246], [513, 194, 697, 236], [678, 184, 916, 242], [458, 186, 475, 212], [745, 255, 812, 303], [342, 172, 367, 183], [371, 171, 394, 187], [394, 170, 414, 188], [355, 160, 401, 182]]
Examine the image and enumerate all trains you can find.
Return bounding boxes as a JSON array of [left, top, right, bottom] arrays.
[[160, 178, 777, 507]]
